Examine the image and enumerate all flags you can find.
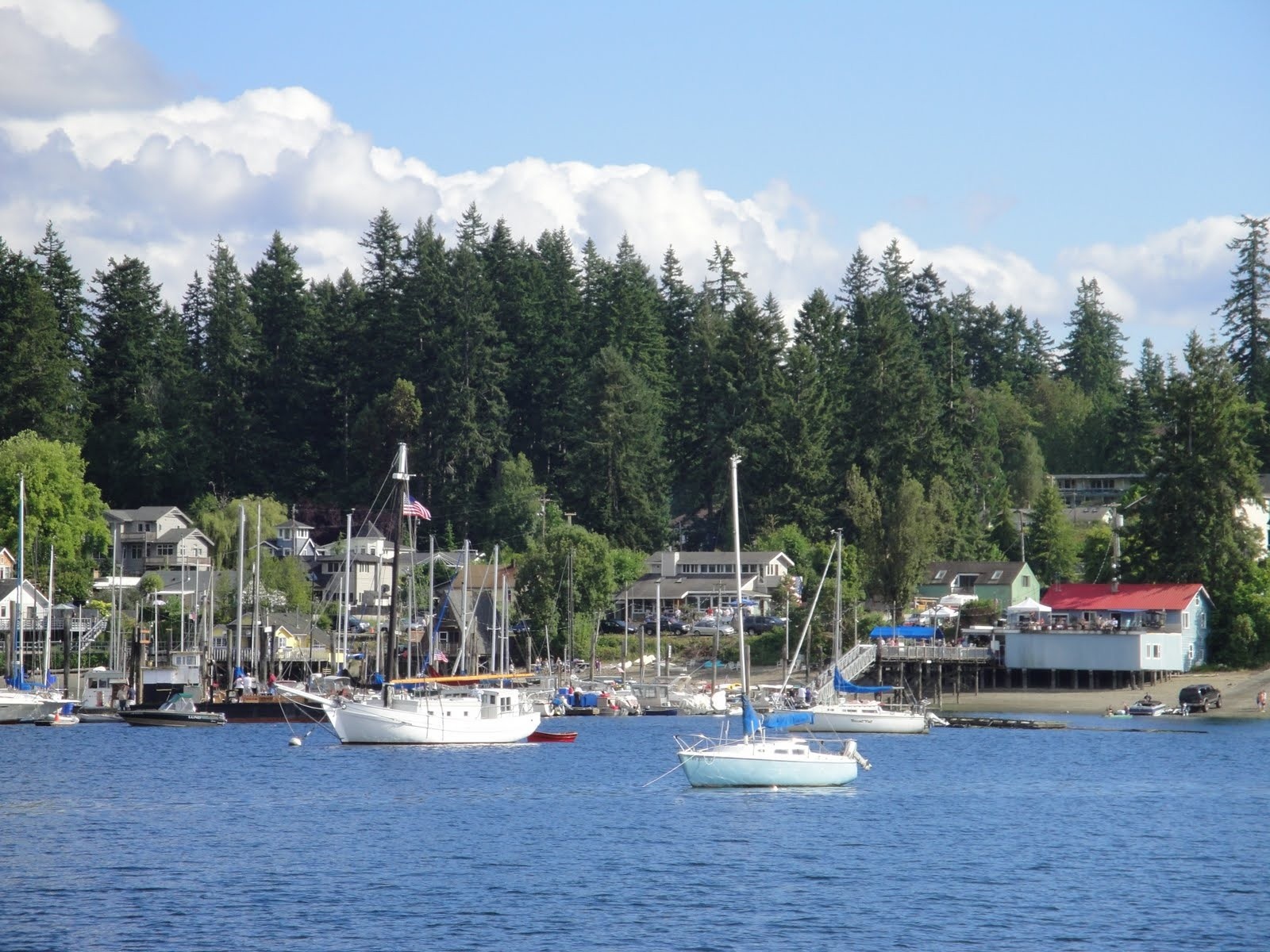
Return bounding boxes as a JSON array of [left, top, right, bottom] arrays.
[[189, 610, 195, 620], [433, 648, 448, 663], [403, 492, 431, 520]]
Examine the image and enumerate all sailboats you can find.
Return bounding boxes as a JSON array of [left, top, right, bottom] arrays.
[[1, 443, 679, 744], [760, 529, 950, 734], [672, 455, 872, 788]]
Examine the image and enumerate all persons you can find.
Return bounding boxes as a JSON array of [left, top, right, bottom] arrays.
[[535, 656, 562, 675], [234, 672, 276, 697], [790, 685, 819, 702], [1257, 690, 1266, 712], [708, 607, 713, 615], [716, 606, 750, 615], [951, 638, 968, 647], [596, 660, 599, 672], [509, 663, 515, 674], [877, 636, 898, 646], [117, 684, 135, 711], [1182, 703, 1191, 716], [1144, 692, 1151, 703], [1100, 618, 1118, 627], [1124, 706, 1129, 715]]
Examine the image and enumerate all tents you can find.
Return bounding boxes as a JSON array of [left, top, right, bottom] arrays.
[[730, 598, 759, 614], [1007, 597, 1052, 626], [919, 605, 959, 621], [869, 626, 945, 646]]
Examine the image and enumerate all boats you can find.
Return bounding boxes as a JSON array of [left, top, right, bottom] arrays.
[[527, 732, 576, 743], [1130, 699, 1166, 717], [47, 714, 80, 726], [117, 694, 227, 729]]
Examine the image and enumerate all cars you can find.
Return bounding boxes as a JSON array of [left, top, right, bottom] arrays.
[[691, 620, 733, 638], [411, 610, 434, 625], [380, 617, 423, 633]]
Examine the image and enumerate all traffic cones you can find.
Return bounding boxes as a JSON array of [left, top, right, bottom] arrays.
[[370, 627, 374, 634]]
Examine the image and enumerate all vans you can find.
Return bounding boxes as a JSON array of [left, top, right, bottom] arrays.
[[744, 616, 784, 636]]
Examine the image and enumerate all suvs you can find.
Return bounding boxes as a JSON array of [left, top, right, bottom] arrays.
[[1179, 684, 1221, 712], [645, 617, 690, 636], [318, 614, 369, 633], [594, 620, 638, 635]]
